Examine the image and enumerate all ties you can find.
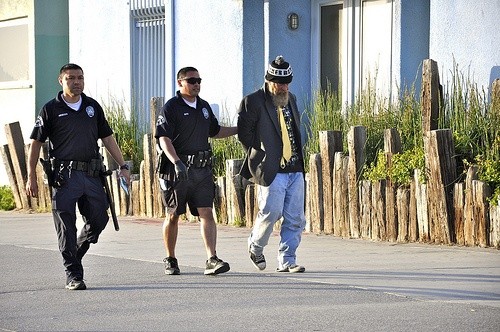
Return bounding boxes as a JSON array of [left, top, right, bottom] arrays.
[[277, 105, 291, 162]]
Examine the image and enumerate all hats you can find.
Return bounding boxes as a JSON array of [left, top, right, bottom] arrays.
[[264, 56, 294, 84]]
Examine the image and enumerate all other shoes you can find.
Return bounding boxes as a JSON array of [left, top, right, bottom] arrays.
[[284, 263, 305, 273], [204, 255, 231, 275], [163, 255, 180, 275], [65, 278, 87, 290], [249, 252, 266, 270]]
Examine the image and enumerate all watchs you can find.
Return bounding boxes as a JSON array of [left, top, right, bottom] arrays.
[[120, 163, 129, 170]]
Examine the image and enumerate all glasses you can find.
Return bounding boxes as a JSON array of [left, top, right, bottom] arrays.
[[181, 77, 202, 84]]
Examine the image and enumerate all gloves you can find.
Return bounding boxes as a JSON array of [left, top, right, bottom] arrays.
[[173, 159, 189, 182]]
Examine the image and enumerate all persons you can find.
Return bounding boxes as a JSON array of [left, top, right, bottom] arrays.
[[237, 55, 306, 272], [154, 66, 238, 274], [26, 64, 130, 289]]
